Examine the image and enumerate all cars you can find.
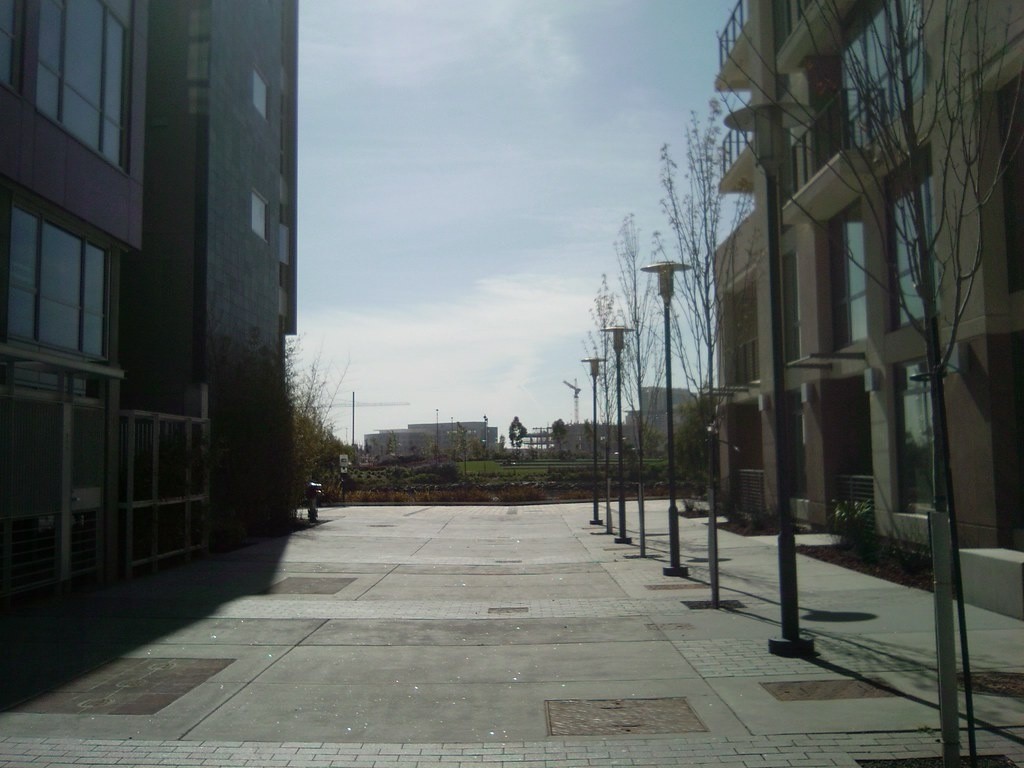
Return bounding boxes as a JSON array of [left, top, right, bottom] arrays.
[[299, 479, 325, 506]]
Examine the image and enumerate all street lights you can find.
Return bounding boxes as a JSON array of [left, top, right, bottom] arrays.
[[600, 324, 638, 545], [719, 99, 818, 656], [581, 358, 608, 526], [643, 261, 688, 578]]
